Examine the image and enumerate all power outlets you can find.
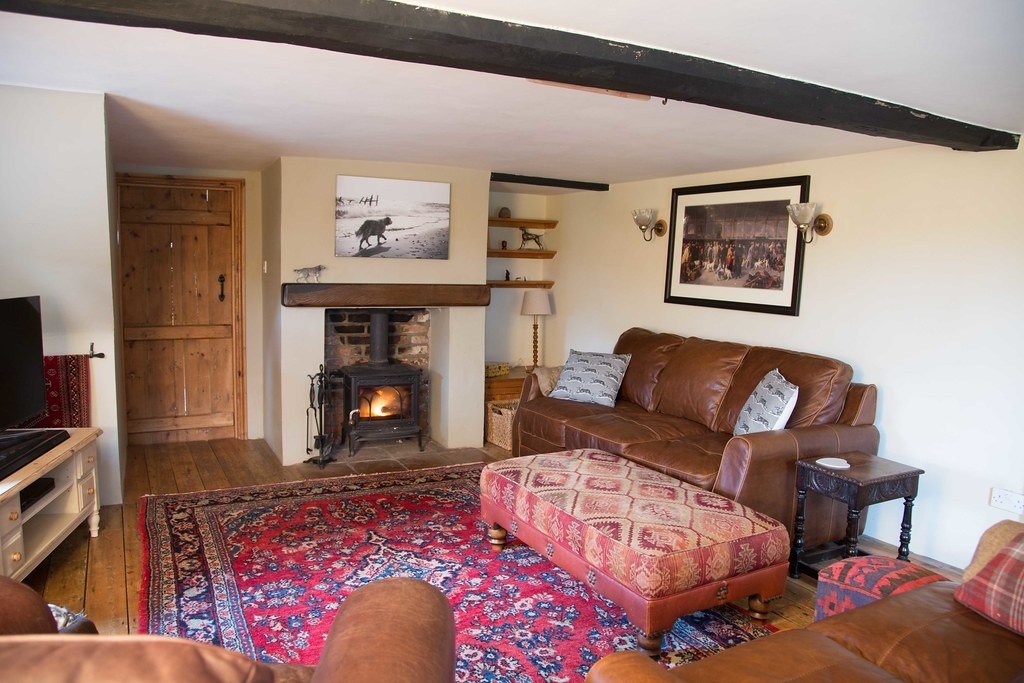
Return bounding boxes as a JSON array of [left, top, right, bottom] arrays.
[[991, 488, 1024, 515]]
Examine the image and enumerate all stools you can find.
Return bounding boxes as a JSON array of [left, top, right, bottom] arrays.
[[815, 553, 950, 623]]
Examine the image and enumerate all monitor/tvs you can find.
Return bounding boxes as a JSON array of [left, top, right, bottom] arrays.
[[0, 296, 48, 449]]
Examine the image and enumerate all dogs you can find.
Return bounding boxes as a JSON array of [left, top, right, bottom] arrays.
[[294, 264, 326, 283], [355, 216, 392, 250], [519, 226, 544, 250]]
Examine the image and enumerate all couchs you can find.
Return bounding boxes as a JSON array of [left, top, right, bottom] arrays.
[[585, 520, 1024, 683], [1, 575, 459, 683], [510, 325, 879, 551]]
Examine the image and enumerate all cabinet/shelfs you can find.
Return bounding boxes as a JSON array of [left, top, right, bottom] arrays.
[[0, 427, 104, 583], [488, 217, 558, 288], [486, 365, 542, 403], [664, 175, 811, 316]]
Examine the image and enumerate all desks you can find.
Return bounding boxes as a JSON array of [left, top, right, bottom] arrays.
[[794, 452, 925, 578]]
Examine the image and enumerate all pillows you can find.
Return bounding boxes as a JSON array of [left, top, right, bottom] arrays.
[[549, 347, 633, 407], [953, 532, 1024, 636], [732, 368, 799, 444]]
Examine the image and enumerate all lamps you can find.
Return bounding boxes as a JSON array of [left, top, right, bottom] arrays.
[[631, 208, 668, 241], [522, 288, 552, 373], [784, 203, 833, 242]]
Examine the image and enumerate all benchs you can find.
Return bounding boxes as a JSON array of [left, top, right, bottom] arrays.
[[480, 448, 791, 662]]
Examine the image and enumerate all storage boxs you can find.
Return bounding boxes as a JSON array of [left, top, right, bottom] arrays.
[[487, 398, 519, 451], [486, 362, 509, 377]]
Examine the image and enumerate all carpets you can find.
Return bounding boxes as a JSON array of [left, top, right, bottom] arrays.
[[139, 458, 782, 683], [10, 354, 90, 428]]
